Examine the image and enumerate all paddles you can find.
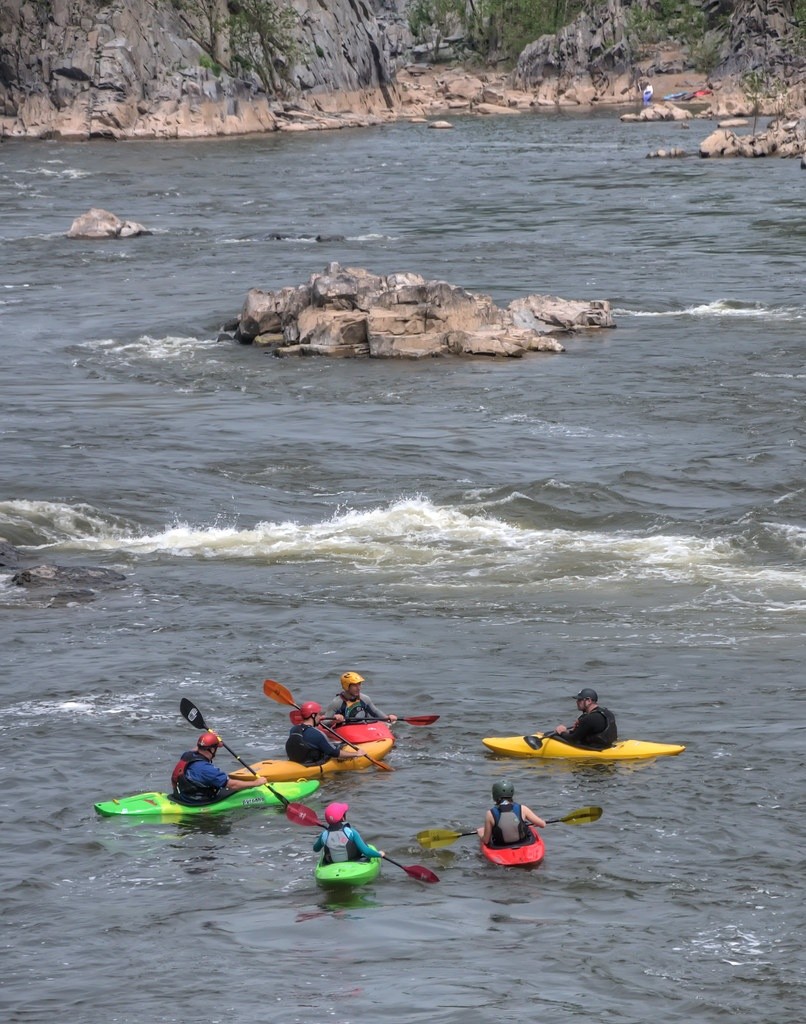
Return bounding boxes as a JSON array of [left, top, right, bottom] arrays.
[[523, 725, 574, 750], [287, 803, 440, 883], [180, 697, 290, 806], [290, 709, 440, 726], [416, 806, 603, 850], [263, 679, 395, 771]]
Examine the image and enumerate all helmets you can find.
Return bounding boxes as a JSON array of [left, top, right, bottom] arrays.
[[340, 672, 365, 691], [325, 802, 349, 824], [300, 701, 321, 719], [197, 733, 223, 747], [492, 780, 514, 801], [572, 688, 598, 701]]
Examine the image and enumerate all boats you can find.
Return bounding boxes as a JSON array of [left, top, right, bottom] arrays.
[[480, 825, 545, 867], [228, 737, 394, 782], [316, 720, 397, 744], [643, 84, 653, 103], [93, 779, 320, 817], [482, 732, 687, 760], [663, 89, 710, 102], [315, 843, 382, 888]]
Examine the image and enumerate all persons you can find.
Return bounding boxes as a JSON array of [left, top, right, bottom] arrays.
[[322, 672, 397, 728], [476, 780, 546, 846], [290, 701, 368, 764], [704, 83, 714, 97], [313, 803, 385, 863], [171, 734, 267, 804], [556, 689, 618, 750]]
